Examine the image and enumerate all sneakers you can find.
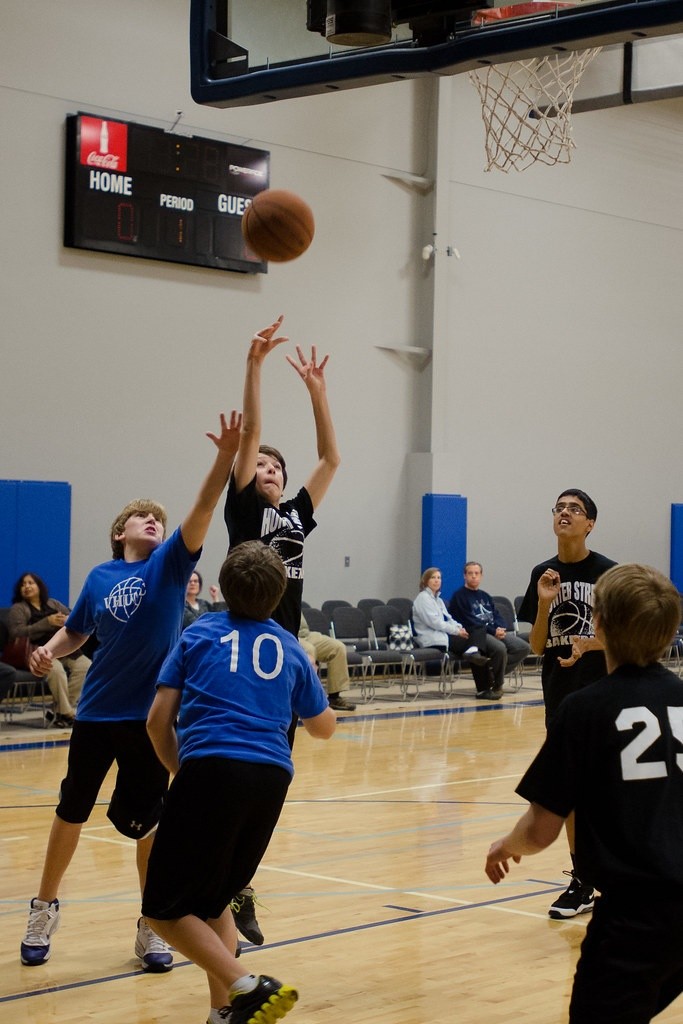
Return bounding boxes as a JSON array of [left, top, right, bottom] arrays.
[[20, 897, 61, 966], [548, 868, 595, 918], [205, 974, 299, 1024], [230, 885, 263, 959], [133, 916, 174, 971]]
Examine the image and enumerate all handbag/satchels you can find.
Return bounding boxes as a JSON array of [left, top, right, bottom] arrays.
[[386, 623, 414, 651], [2, 635, 38, 671]]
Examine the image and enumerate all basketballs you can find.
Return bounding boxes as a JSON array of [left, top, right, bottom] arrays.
[[241, 190, 315, 262]]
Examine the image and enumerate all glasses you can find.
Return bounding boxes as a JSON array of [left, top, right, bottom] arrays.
[[552, 506, 591, 520]]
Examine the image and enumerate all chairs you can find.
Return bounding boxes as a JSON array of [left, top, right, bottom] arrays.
[[658, 626, 683, 677], [0, 608, 69, 729], [302, 597, 545, 705]]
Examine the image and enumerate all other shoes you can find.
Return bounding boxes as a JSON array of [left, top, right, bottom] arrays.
[[475, 688, 501, 700], [499, 683, 515, 693], [463, 652, 487, 666], [327, 697, 355, 710], [492, 686, 504, 698], [46, 710, 75, 728]]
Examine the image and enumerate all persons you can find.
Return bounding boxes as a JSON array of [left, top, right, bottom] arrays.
[[7, 571, 356, 729], [18, 314, 341, 1024], [486, 488, 683, 1024], [413, 560, 531, 701]]
